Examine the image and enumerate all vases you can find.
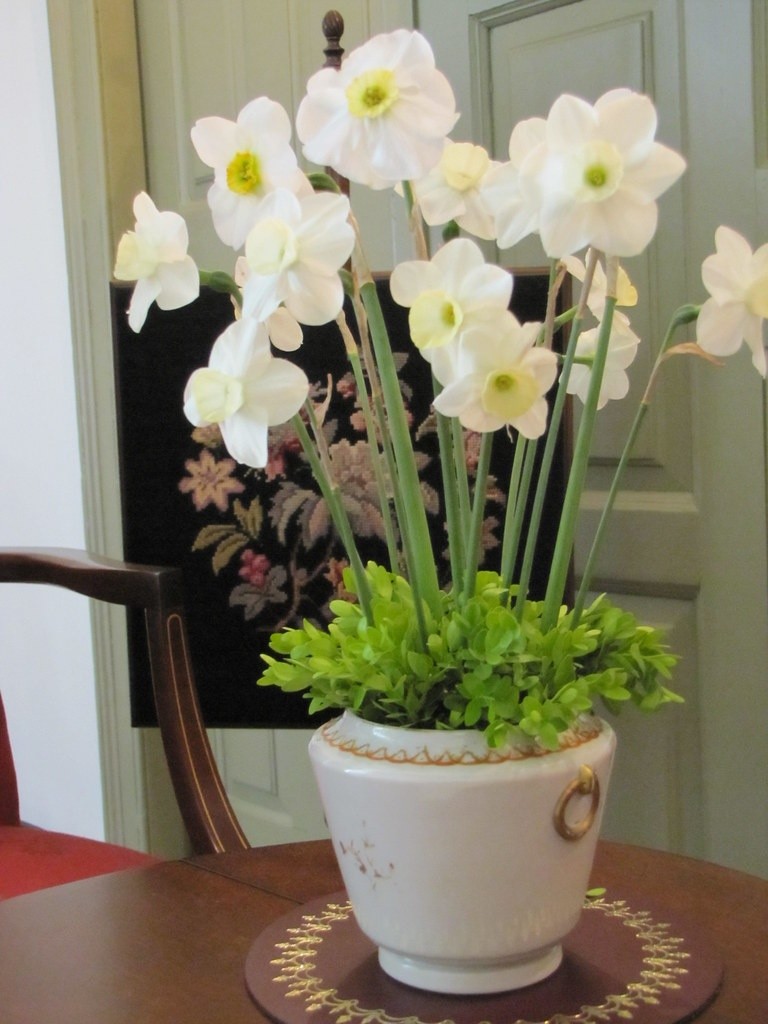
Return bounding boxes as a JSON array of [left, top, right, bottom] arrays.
[[313, 710, 620, 998]]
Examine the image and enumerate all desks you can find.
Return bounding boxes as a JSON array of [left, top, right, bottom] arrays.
[[0, 836, 768, 1024]]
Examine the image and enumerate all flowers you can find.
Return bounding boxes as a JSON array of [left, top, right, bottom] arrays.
[[110, 29, 768, 759]]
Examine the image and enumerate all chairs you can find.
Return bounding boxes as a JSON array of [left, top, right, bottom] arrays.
[[0, 547, 250, 906]]
